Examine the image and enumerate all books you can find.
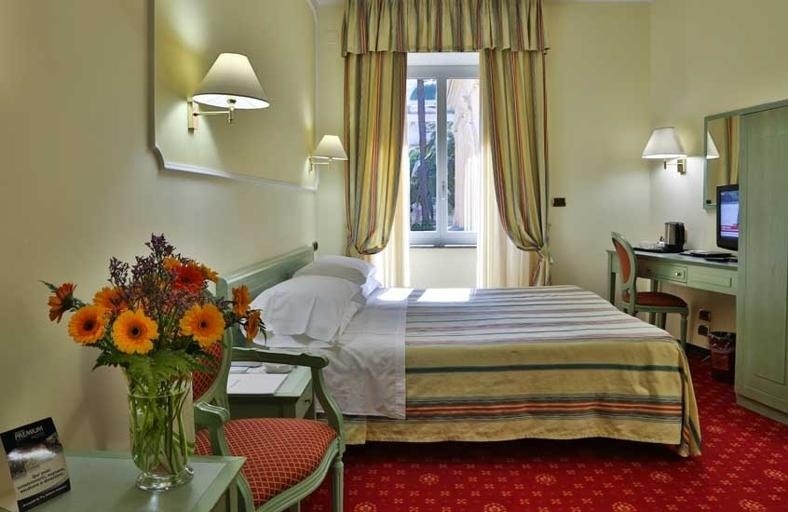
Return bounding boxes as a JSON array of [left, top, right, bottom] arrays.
[[227, 373, 288, 396]]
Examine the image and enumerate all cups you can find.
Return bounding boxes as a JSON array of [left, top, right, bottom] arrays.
[[641, 241, 665, 249]]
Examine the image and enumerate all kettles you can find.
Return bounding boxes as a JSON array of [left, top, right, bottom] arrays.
[[664, 220, 685, 251]]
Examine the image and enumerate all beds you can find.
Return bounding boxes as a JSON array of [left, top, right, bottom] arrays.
[[210, 237, 706, 462]]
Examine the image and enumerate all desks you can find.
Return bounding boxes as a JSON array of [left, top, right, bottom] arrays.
[[1, 445, 257, 511], [605, 247, 738, 325]]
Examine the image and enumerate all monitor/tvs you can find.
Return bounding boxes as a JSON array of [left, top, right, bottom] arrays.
[[715, 183, 739, 251]]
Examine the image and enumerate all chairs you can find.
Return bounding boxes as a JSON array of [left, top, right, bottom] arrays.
[[172, 283, 348, 510], [607, 230, 690, 354]]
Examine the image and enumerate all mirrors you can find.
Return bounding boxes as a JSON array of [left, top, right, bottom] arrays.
[[701, 98, 788, 209]]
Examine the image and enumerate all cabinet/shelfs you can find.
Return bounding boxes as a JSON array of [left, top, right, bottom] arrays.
[[733, 97, 788, 426]]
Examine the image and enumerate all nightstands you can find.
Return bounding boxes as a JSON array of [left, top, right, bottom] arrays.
[[227, 363, 322, 426]]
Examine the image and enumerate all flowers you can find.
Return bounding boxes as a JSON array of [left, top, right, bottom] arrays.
[[37, 232, 270, 483]]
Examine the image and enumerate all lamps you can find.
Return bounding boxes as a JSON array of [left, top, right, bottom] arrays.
[[706, 131, 720, 160], [183, 51, 272, 131], [306, 134, 348, 172], [641, 127, 687, 174]]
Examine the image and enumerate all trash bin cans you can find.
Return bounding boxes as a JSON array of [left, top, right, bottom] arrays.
[[708, 331, 735, 382]]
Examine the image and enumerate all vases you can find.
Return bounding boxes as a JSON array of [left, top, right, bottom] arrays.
[[119, 359, 198, 494]]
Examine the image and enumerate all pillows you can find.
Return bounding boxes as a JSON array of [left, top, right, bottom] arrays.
[[242, 254, 384, 347]]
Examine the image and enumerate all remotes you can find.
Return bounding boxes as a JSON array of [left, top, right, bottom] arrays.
[[705, 256, 738, 263]]
